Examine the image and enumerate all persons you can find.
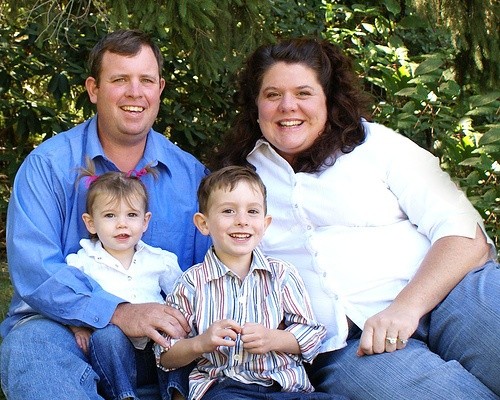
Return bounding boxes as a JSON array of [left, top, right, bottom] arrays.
[[1, 31, 215, 400], [220, 37, 500, 400], [154, 166, 351, 400], [65, 157, 200, 399]]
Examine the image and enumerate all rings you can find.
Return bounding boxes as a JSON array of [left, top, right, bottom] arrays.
[[386, 337, 409, 344]]
